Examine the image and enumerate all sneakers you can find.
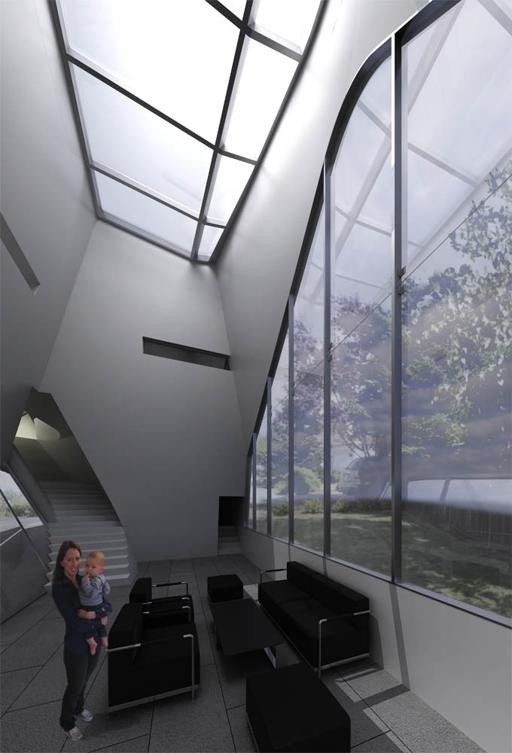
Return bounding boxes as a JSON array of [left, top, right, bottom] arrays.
[[79, 709, 93, 722], [65, 727, 82, 741]]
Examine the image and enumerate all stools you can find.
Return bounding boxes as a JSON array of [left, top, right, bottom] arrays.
[[207, 574, 243, 602], [245, 661, 350, 751], [244, 655, 280, 694]]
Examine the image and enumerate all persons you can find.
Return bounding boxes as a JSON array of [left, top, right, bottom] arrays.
[[51, 540, 112, 744]]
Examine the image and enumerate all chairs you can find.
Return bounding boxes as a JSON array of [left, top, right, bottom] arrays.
[[107, 576, 201, 715]]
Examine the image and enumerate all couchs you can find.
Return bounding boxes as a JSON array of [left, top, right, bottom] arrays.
[[257, 561, 372, 677]]
